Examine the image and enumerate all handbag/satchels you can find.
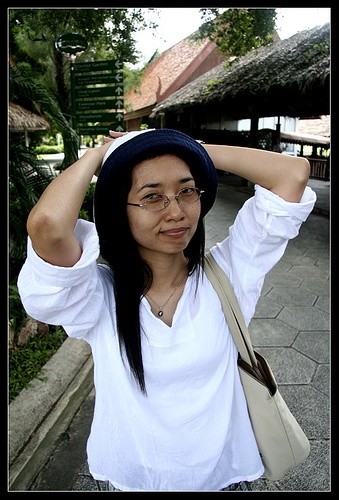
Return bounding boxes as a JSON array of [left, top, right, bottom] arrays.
[[199, 250, 311, 481]]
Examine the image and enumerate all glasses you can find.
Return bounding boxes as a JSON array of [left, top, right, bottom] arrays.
[[125, 187, 204, 213]]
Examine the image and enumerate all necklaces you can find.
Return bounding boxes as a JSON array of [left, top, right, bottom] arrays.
[[143, 280, 183, 316]]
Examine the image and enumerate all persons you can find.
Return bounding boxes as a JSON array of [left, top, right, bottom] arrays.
[[17, 128, 317, 491]]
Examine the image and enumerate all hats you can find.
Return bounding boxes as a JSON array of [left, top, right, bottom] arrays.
[[92, 128, 216, 236]]
